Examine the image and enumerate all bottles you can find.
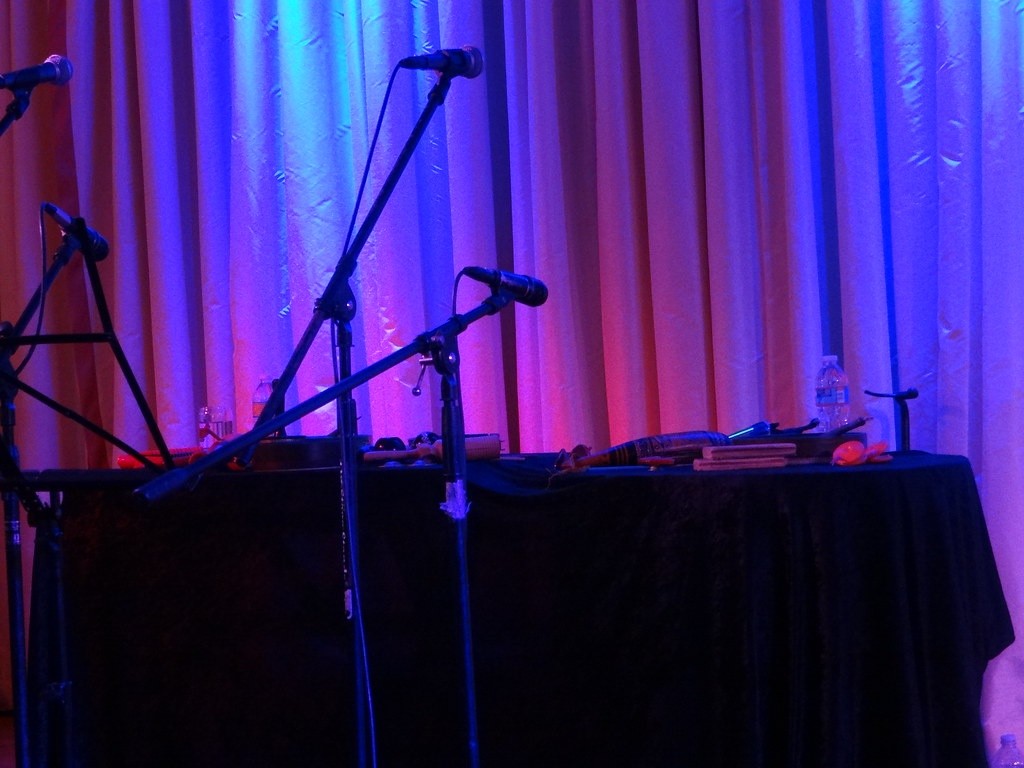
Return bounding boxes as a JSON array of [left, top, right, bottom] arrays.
[[815, 356, 850, 433], [252, 375, 274, 437]]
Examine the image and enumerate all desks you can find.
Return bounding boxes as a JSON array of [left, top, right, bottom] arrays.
[[26, 449, 1017, 768]]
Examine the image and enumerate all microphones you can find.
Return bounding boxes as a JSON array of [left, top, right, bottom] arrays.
[[44, 202, 109, 262], [462, 266, 549, 307], [400, 46, 485, 79], [0, 54, 74, 90]]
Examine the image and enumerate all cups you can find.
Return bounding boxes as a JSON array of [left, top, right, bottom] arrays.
[[197, 405, 233, 451]]
